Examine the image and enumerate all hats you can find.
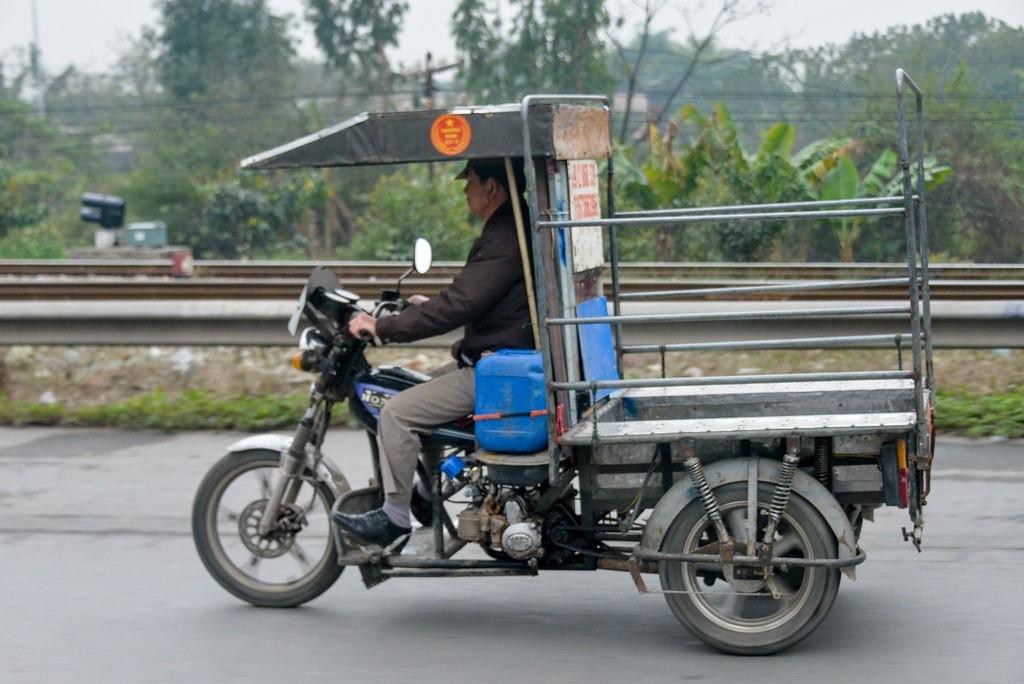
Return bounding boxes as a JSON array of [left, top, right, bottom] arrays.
[[454, 156, 524, 182]]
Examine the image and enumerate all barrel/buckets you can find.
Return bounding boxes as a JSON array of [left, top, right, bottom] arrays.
[[474, 348, 548, 452]]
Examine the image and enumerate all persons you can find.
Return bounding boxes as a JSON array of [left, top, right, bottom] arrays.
[[331, 157, 540, 554]]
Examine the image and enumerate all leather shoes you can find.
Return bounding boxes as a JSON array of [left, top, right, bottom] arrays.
[[329, 508, 412, 555], [410, 481, 434, 526]]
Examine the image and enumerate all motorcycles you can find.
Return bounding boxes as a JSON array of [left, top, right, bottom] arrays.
[[191, 66, 939, 655]]
